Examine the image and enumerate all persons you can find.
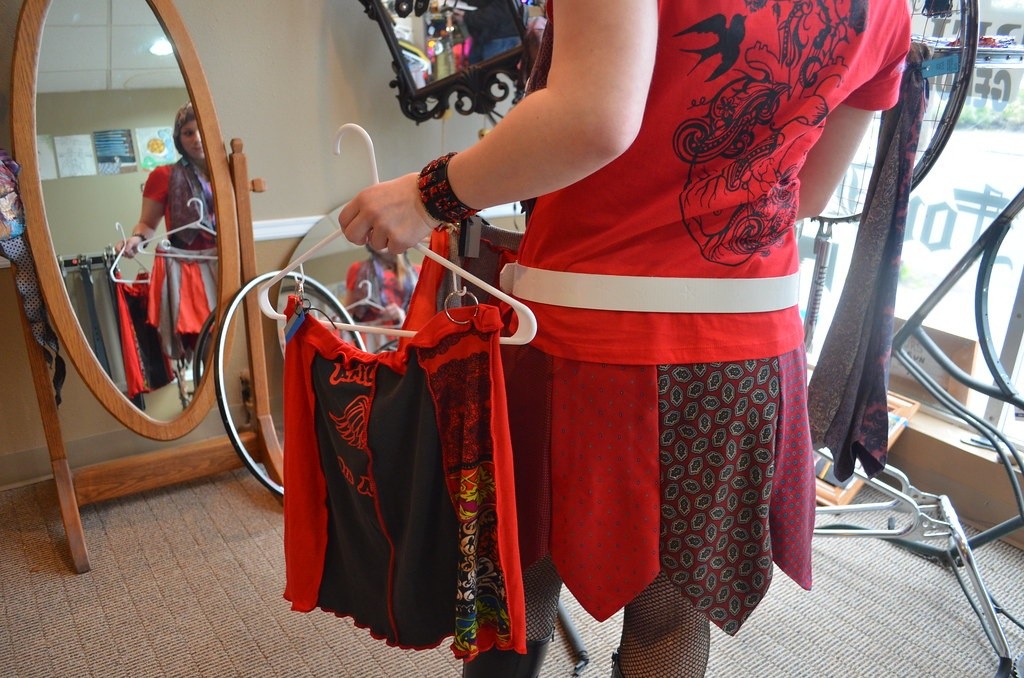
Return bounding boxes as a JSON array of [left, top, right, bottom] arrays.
[[339, 0, 911, 678], [462, 0, 520, 64], [115, 100, 218, 366], [344, 243, 422, 323]]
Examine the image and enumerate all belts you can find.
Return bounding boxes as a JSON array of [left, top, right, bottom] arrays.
[[498, 260, 800, 314]]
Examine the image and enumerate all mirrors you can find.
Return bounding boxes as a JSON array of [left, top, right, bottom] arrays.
[[376, 0, 523, 90], [276, 201, 432, 359], [8, 0, 286, 573]]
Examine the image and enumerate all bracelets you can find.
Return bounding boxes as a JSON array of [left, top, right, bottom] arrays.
[[134, 233, 148, 248], [419, 151, 481, 225]]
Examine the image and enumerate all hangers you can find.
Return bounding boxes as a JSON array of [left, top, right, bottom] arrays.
[[257, 123, 537, 344], [137, 197, 221, 260], [318, 280, 403, 329], [109, 222, 152, 283]]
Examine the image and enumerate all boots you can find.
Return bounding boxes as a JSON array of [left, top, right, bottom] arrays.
[[610, 646, 625, 678], [463, 624, 555, 678]]
